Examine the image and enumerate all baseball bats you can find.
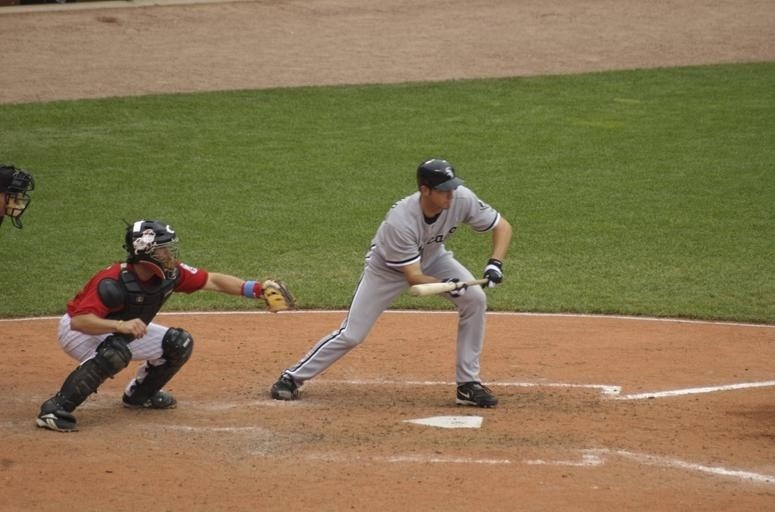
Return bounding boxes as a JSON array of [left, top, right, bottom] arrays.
[[410, 277, 489, 297]]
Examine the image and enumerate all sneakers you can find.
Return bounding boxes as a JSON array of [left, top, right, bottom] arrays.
[[122, 392, 177, 410], [272, 372, 299, 402], [456, 381, 498, 407], [35, 407, 78, 433]]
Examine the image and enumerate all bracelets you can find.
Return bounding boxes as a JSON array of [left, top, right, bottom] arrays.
[[240, 280, 263, 299]]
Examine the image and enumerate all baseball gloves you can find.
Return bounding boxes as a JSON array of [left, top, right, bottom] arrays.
[[260, 279, 294, 312]]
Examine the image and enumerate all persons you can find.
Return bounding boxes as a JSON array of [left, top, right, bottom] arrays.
[[0, 165, 35, 229], [35, 220, 296, 433], [270, 158, 512, 410]]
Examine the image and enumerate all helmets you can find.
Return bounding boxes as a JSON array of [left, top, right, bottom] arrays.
[[417, 157, 465, 194], [122, 218, 177, 282], [0, 167, 33, 197]]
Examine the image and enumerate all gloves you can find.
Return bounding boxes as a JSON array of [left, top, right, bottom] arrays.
[[482, 257, 506, 291], [441, 275, 468, 297]]
